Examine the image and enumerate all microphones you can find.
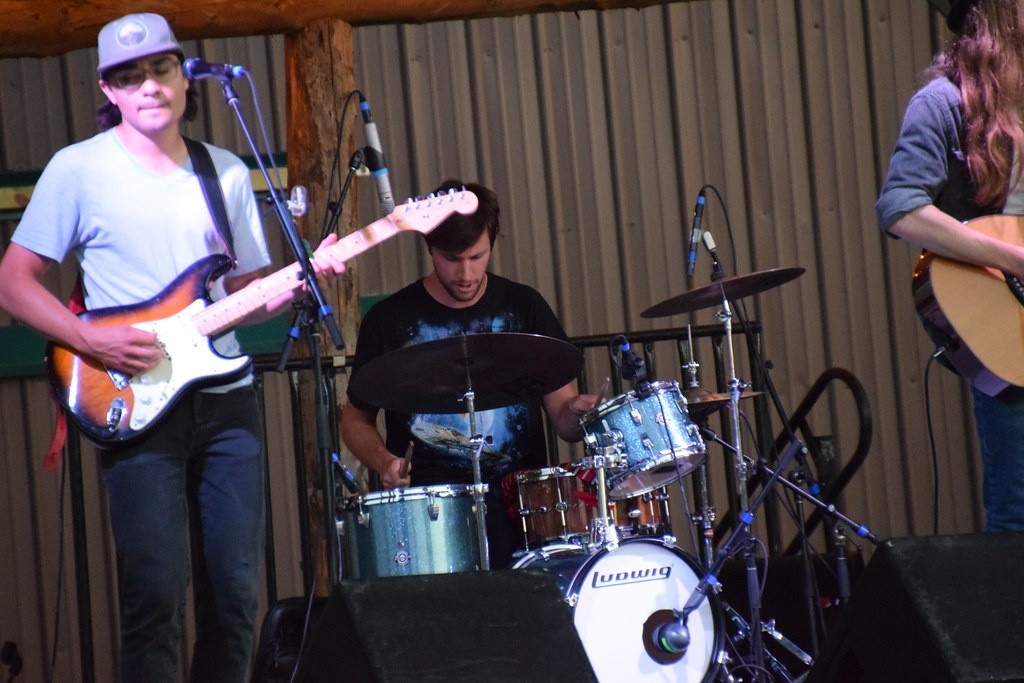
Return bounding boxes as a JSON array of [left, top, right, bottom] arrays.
[[360, 93, 395, 216], [620, 339, 636, 380], [183, 57, 246, 80], [687, 191, 706, 276], [331, 452, 362, 494], [654, 624, 690, 652]]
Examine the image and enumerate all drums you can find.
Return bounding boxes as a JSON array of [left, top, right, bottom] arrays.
[[511, 535, 728, 683], [335, 483, 483, 581], [513, 458, 666, 547], [577, 379, 711, 502]]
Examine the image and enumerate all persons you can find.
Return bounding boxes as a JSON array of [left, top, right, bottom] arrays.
[[340, 179, 615, 572], [877, 0, 1024, 534], [0, 11, 346, 683]]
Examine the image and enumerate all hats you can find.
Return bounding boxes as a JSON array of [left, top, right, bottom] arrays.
[[96, 12, 185, 79]]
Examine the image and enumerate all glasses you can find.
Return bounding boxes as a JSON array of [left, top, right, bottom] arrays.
[[106, 57, 181, 89]]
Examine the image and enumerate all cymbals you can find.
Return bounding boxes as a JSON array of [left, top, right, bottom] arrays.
[[349, 332, 586, 415], [639, 262, 808, 323], [682, 387, 767, 416]]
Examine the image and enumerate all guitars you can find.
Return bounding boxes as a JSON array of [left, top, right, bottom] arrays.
[[909, 213, 1024, 405], [40, 184, 481, 455]]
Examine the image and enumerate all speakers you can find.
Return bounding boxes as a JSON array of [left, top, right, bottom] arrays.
[[794, 529, 1024, 683], [249, 593, 326, 683], [295, 565, 600, 683]]
